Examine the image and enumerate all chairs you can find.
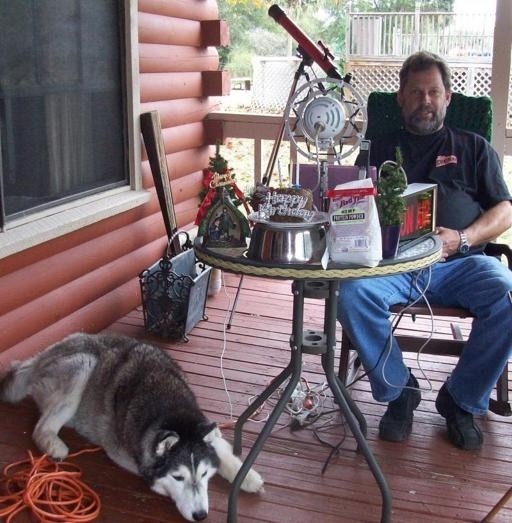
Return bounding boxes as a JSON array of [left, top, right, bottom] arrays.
[[338, 89, 510, 419]]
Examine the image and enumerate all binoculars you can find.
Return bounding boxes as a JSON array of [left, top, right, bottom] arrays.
[[268, 3, 352, 86]]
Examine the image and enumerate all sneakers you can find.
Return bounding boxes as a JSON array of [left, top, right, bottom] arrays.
[[436, 384, 483, 449], [379, 372, 421, 441]]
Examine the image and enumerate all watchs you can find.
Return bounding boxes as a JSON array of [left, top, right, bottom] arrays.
[[456, 228, 470, 257]]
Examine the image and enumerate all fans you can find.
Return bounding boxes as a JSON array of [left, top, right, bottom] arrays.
[[285, 76, 368, 212]]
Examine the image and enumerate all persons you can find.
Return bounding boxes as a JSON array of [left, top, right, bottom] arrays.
[[336, 50, 512, 450]]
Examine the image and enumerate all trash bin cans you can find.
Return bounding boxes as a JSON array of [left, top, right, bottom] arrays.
[[244, 79, 250, 90]]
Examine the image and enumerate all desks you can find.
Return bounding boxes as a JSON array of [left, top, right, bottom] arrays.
[[196, 233, 445, 521]]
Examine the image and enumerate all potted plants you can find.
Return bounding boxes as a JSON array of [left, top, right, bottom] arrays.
[[374, 146, 408, 259]]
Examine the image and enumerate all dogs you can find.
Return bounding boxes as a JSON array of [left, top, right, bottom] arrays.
[[0, 332, 265, 523]]
